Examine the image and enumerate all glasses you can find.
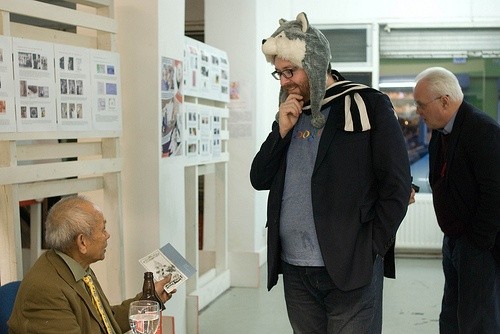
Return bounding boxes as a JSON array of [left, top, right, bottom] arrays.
[[415, 94, 448, 110], [271, 65, 299, 80]]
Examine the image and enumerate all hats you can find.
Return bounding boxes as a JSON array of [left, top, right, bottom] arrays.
[[261, 11, 332, 128]]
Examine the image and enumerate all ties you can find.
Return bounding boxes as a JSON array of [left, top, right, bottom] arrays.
[[82, 275, 116, 334]]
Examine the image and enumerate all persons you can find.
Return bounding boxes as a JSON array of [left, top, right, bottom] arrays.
[[250, 12, 420, 334], [7, 195, 178, 334], [412, 67, 500, 334]]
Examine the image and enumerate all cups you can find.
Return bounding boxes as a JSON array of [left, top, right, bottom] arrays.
[[128, 300, 160, 334]]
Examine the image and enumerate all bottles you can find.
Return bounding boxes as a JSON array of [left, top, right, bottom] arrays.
[[139, 271, 161, 306]]
